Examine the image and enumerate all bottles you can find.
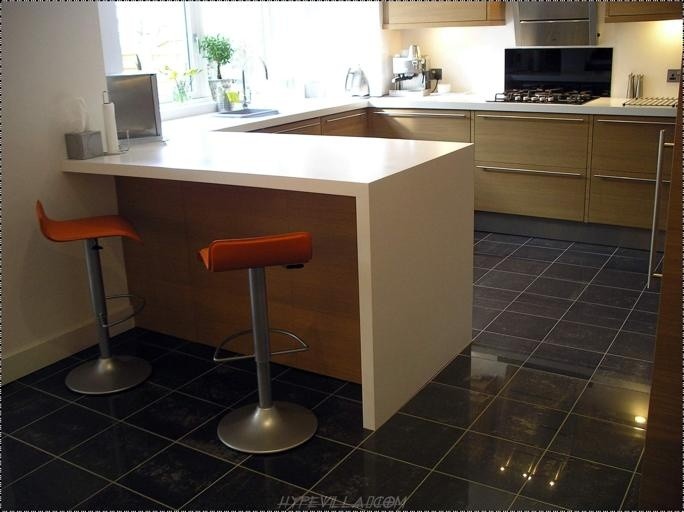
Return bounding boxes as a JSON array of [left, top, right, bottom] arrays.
[[626, 72, 642, 98]]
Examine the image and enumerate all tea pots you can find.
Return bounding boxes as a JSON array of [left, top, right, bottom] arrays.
[[344, 65, 370, 99]]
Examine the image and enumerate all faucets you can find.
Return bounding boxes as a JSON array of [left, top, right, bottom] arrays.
[[241, 54, 270, 107]]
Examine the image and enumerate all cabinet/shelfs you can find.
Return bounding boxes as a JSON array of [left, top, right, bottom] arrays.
[[604, 2, 682, 23], [381, 1, 507, 28], [584, 114, 676, 232], [367, 110, 474, 234], [470, 111, 589, 222], [236, 111, 367, 138]]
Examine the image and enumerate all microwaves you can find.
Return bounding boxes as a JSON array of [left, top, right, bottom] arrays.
[[105, 71, 162, 138]]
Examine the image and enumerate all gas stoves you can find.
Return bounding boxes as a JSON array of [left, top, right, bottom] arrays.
[[494, 88, 599, 106]]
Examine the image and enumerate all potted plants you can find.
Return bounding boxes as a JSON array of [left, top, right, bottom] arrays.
[[167, 66, 201, 103], [201, 35, 238, 102]]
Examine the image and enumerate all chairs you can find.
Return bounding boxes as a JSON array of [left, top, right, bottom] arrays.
[[36, 200, 150, 394], [199, 230, 319, 453]]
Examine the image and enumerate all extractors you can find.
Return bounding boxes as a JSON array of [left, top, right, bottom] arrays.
[[513, 0, 599, 45]]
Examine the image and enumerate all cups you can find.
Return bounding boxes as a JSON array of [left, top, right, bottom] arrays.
[[111, 127, 130, 152], [437, 83, 450, 94], [227, 87, 243, 108]]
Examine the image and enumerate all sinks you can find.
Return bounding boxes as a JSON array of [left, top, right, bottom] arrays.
[[220, 108, 267, 114]]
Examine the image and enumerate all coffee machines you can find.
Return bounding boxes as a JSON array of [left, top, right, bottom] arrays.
[[388, 45, 431, 97]]
